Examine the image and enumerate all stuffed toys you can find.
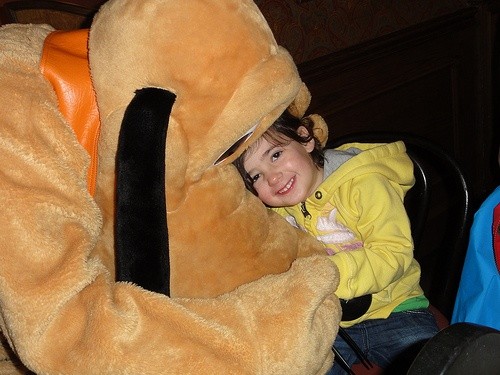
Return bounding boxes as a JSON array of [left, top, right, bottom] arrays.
[[1, 0, 343, 375]]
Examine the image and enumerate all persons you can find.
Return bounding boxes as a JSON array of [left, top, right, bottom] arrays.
[[225, 108, 443, 375], [450, 185, 500, 333]]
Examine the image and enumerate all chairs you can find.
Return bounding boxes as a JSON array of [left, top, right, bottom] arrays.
[[334, 130, 468, 287]]
